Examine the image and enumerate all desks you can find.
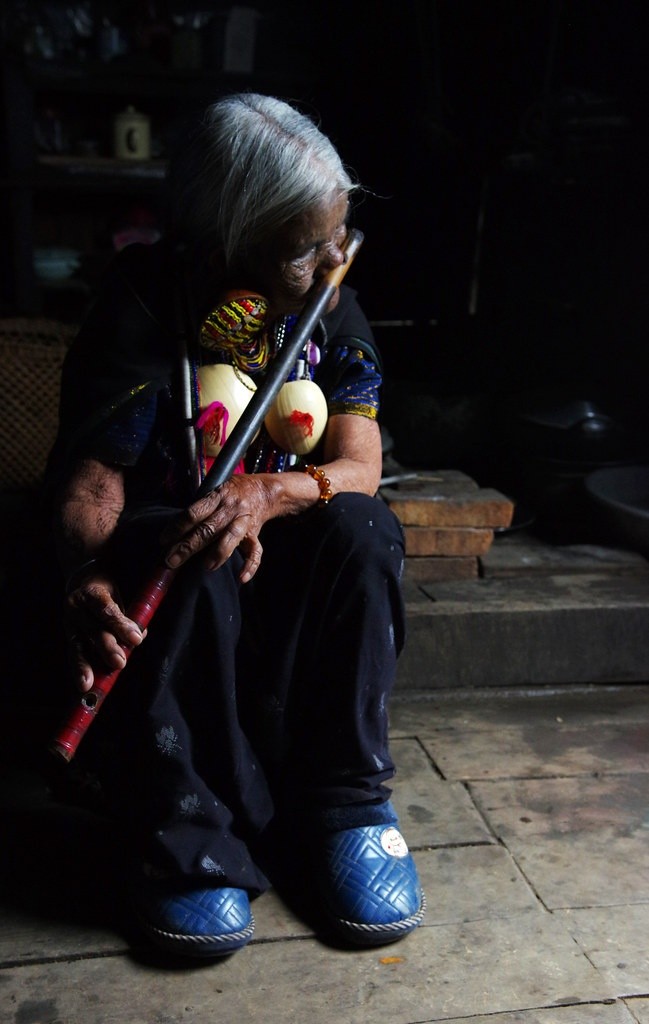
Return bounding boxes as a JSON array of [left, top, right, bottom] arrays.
[[34, 156, 174, 179]]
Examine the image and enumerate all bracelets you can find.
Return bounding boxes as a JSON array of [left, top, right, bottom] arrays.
[[296, 460, 333, 508]]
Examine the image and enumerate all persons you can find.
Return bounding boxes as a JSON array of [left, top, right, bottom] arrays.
[[40, 91, 428, 952]]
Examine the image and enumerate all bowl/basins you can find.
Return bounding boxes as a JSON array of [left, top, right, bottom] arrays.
[[584, 466, 649, 551]]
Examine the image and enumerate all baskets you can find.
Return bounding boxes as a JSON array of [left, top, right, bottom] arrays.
[[1, 320, 79, 482]]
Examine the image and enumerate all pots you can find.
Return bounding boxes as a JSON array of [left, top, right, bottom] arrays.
[[512, 397, 626, 459]]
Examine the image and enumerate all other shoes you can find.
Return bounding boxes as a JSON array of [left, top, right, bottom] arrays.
[[136, 865, 256, 959], [313, 800, 426, 948]]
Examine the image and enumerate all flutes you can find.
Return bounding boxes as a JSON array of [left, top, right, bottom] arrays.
[[42, 227, 374, 772]]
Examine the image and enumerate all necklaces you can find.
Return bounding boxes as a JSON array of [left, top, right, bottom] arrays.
[[273, 451, 284, 472], [251, 448, 263, 473], [301, 337, 320, 380], [265, 453, 274, 473]]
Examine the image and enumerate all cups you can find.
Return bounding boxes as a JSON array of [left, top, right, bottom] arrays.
[[113, 105, 150, 160]]
